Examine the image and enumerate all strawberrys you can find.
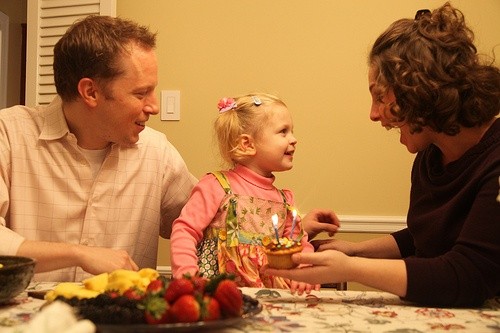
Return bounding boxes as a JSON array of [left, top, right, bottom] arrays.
[[98, 267, 243, 325]]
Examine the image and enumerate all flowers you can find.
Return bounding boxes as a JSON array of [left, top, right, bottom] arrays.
[[217, 97, 237, 112]]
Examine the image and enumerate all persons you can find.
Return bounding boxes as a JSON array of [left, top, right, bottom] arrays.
[[259, 0, 500, 308], [0, 16, 340, 284], [169, 92, 320, 296]]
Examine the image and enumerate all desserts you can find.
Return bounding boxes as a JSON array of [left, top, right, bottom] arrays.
[[264, 237, 302, 270]]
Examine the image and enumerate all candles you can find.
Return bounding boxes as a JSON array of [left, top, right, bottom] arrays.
[[288, 209, 298, 241], [271, 214, 282, 245]]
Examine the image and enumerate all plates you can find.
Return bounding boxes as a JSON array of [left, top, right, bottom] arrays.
[[96, 294, 263, 333]]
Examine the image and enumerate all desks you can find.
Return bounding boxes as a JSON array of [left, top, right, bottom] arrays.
[[0, 283, 500, 333]]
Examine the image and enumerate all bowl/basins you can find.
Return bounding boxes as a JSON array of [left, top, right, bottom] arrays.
[[0, 255, 36, 303]]
